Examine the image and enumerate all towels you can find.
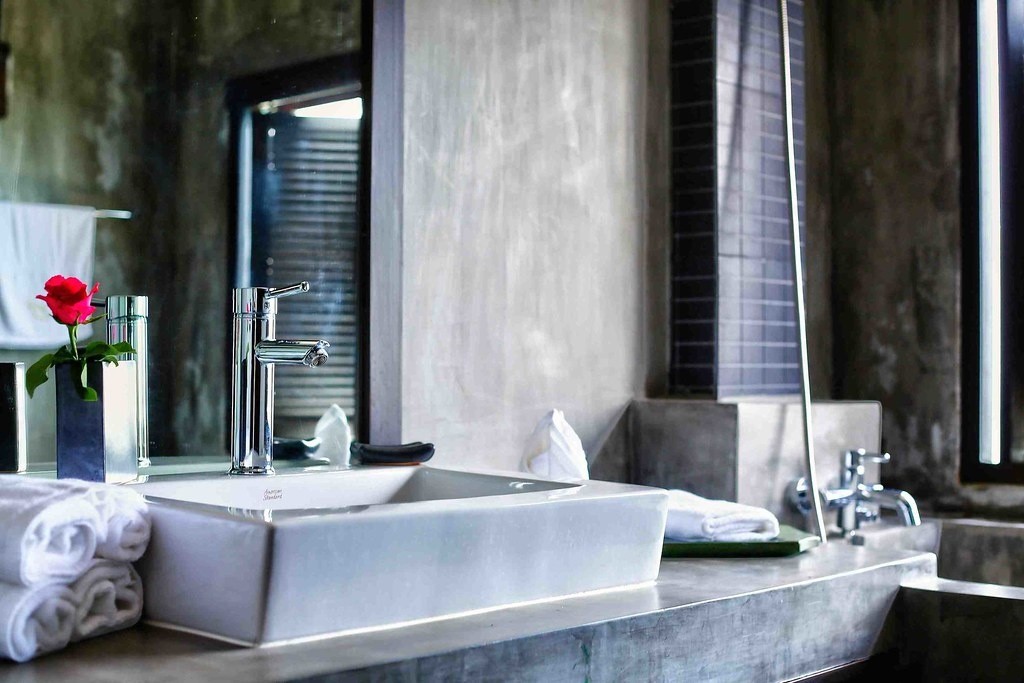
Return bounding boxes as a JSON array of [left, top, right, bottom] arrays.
[[0, 473, 154, 664], [663, 487, 781, 543], [0, 202, 96, 364]]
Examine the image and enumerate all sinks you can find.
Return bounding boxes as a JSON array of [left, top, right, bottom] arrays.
[[128, 460, 676, 648]]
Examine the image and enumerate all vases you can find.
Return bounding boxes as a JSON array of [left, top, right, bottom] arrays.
[[55, 360, 138, 485]]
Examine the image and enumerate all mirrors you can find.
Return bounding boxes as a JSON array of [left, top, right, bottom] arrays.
[[0, 0, 406, 479]]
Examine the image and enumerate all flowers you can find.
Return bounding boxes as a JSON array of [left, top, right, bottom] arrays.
[[25, 274, 136, 400]]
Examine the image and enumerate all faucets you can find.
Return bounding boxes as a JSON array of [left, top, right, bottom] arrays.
[[839, 447, 922, 533], [90, 293, 158, 467], [230, 277, 330, 474]]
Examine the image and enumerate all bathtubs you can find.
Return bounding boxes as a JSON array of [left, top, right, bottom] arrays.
[[849, 514, 1024, 683]]
